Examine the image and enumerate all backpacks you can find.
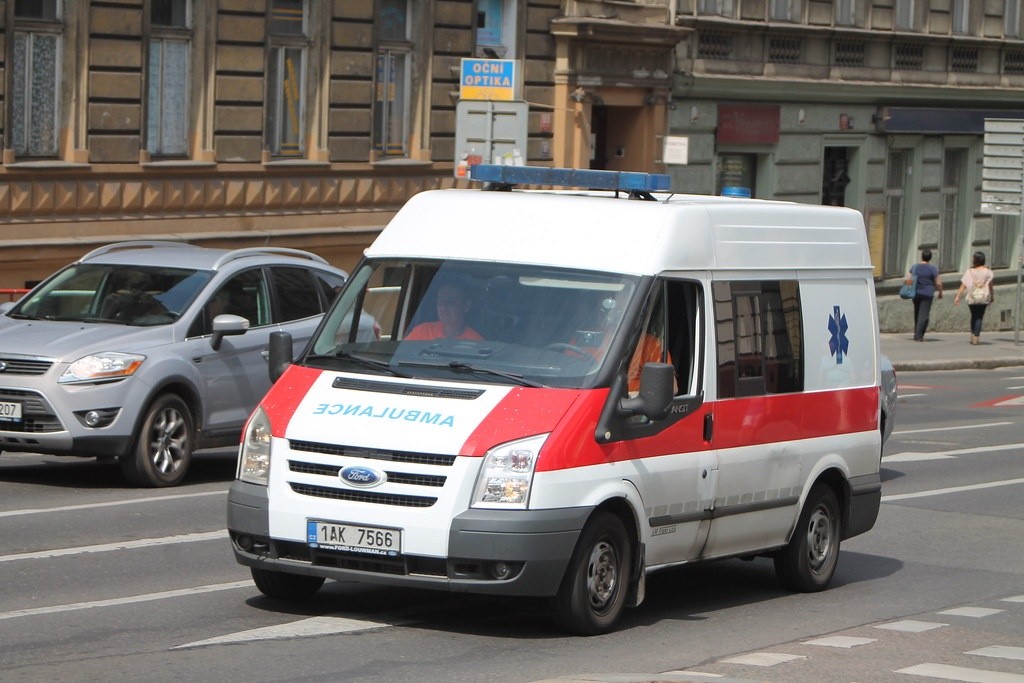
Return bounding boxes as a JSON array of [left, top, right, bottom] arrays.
[[969, 269, 991, 305]]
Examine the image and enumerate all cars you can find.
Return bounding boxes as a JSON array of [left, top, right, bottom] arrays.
[[880, 352, 898, 450]]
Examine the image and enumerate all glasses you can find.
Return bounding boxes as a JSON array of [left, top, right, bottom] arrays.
[[211, 297, 220, 303]]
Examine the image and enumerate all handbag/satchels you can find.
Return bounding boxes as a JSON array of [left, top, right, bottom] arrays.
[[900, 263, 917, 299]]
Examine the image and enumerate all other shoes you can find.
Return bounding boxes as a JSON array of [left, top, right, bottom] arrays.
[[914, 336, 923, 341], [971, 337, 980, 345]]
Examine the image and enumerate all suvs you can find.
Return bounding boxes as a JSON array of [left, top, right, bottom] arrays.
[[0, 241, 384, 487]]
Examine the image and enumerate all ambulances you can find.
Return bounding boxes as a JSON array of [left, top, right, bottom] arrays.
[[224, 165, 882, 638]]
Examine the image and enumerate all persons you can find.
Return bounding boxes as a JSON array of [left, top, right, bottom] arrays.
[[563, 292, 680, 399], [954, 251, 995, 344], [403, 279, 484, 341], [908, 249, 944, 340]]
